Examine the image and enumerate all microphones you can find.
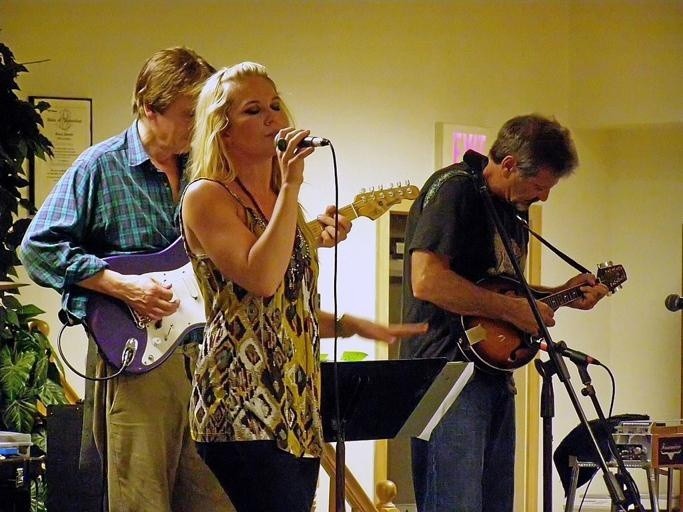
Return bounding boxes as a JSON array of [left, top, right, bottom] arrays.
[[297, 137, 329, 147], [664, 294, 682, 311], [529, 337, 598, 363]]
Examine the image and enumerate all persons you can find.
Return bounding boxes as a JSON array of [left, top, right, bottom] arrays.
[[395, 113, 612, 512], [174, 60, 429, 511], [14, 43, 355, 510]]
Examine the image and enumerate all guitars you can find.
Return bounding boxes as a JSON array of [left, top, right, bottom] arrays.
[[86, 180, 419, 373]]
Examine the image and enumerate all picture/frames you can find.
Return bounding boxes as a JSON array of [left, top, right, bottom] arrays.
[[27, 96, 93, 215]]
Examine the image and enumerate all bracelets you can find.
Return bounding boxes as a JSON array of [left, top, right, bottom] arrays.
[[336, 311, 347, 338]]
